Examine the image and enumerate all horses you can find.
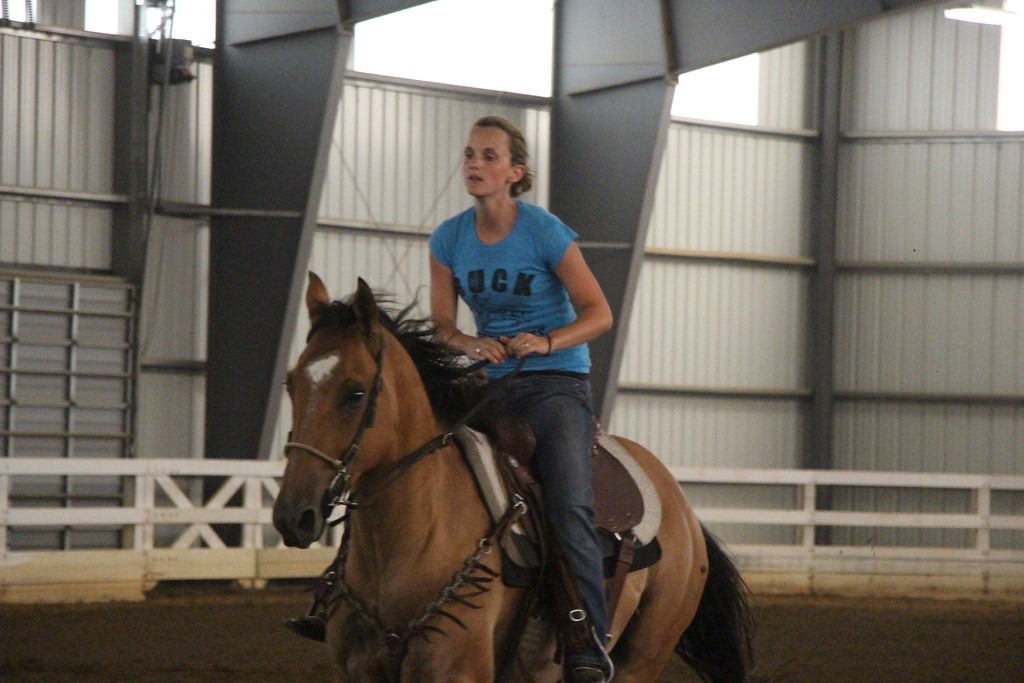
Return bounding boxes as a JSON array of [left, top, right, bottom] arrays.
[[272, 273, 764, 683]]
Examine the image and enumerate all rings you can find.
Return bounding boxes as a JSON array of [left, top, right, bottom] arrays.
[[524, 343, 528, 347], [475, 347, 482, 352]]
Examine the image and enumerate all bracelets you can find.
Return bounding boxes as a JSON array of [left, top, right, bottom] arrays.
[[541, 334, 552, 357]]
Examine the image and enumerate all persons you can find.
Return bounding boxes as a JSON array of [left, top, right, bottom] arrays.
[[429, 117, 613, 683]]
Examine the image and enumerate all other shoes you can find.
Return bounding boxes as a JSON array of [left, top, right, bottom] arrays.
[[563, 665, 605, 681], [286, 615, 328, 642]]
